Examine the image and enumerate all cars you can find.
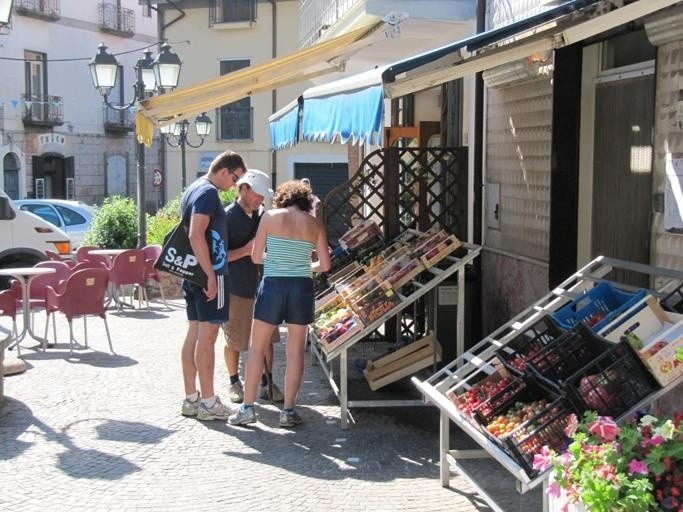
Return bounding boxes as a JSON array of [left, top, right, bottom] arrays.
[[13, 199, 97, 249]]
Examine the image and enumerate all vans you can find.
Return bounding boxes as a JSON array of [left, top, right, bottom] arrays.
[[0, 190, 74, 287]]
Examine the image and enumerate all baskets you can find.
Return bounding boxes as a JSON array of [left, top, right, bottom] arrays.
[[473, 280, 661, 479], [313, 233, 393, 296]]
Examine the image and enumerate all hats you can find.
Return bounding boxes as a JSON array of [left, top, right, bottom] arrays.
[[237, 169, 274, 197]]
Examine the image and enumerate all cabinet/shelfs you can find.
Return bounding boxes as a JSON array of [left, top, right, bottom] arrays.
[[301, 228, 481, 425], [410, 255, 681, 492]]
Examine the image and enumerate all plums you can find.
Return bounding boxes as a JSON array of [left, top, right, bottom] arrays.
[[321, 316, 354, 344]]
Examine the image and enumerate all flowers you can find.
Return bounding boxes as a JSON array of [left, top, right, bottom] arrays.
[[534, 414, 683, 512]]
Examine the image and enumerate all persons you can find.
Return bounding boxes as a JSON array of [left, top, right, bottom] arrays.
[[219, 166, 288, 405], [224, 177, 333, 431], [298, 177, 323, 219], [174, 147, 249, 424]]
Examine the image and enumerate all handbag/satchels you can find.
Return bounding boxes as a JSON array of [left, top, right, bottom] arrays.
[[152, 216, 213, 289]]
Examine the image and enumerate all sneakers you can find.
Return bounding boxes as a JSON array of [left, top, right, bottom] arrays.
[[182, 380, 303, 427]]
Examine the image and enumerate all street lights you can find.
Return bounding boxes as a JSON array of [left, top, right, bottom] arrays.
[[158, 113, 213, 192], [88, 41, 183, 249]]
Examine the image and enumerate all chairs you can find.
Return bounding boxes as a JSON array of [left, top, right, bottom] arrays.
[[0, 244, 162, 362]]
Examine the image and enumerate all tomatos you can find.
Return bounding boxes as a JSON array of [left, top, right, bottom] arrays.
[[458, 348, 569, 463]]
